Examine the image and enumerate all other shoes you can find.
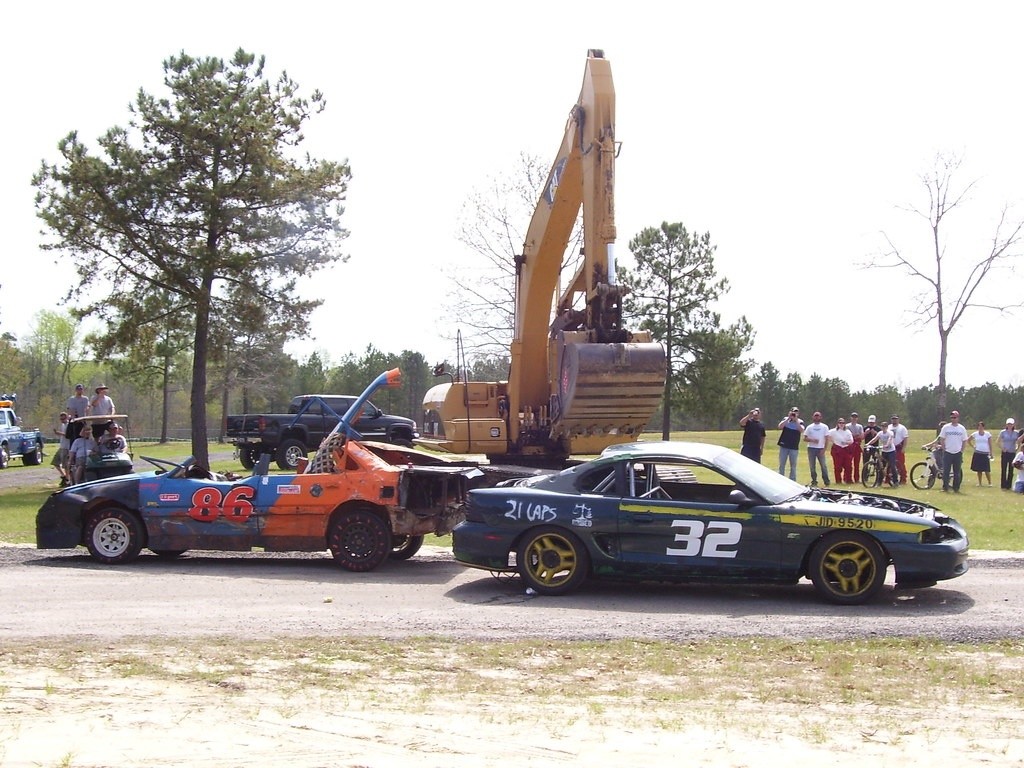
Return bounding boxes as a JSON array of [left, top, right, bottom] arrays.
[[796, 476, 1003, 491], [59, 475, 70, 488]]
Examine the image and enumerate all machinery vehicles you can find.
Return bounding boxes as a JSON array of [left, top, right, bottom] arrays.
[[408, 48, 696, 484]]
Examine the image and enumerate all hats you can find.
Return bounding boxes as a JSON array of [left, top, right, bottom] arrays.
[[110, 422, 118, 429], [837, 417, 845, 423], [852, 412, 858, 417], [868, 415, 877, 423], [1005, 418, 1015, 424], [891, 414, 898, 418], [814, 411, 820, 417], [791, 407, 798, 412], [950, 410, 959, 416], [95, 383, 108, 394], [76, 384, 83, 389], [881, 422, 888, 427]]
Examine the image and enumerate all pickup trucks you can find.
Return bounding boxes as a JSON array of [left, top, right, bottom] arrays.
[[226, 393, 421, 469], [0, 394, 45, 470]]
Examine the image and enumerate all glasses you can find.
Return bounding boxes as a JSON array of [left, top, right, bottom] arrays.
[[950, 417, 958, 419]]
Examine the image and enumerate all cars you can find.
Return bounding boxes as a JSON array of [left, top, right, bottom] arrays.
[[446, 442, 969, 605], [28, 366, 473, 571]]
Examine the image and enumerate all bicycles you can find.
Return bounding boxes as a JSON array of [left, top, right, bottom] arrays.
[[909, 447, 963, 490], [861, 443, 903, 488]]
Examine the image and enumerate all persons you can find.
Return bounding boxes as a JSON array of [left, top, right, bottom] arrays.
[[829, 418, 854, 484], [863, 414, 881, 482], [803, 412, 830, 486], [846, 413, 863, 483], [776, 407, 805, 481], [940, 411, 967, 492], [740, 408, 766, 464], [864, 422, 899, 487], [922, 421, 948, 475], [50, 384, 128, 485], [884, 414, 909, 486], [967, 423, 993, 486], [996, 418, 1024, 492]]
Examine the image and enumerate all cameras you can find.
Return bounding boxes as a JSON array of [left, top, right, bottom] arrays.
[[752, 411, 757, 415], [792, 413, 795, 417]]
